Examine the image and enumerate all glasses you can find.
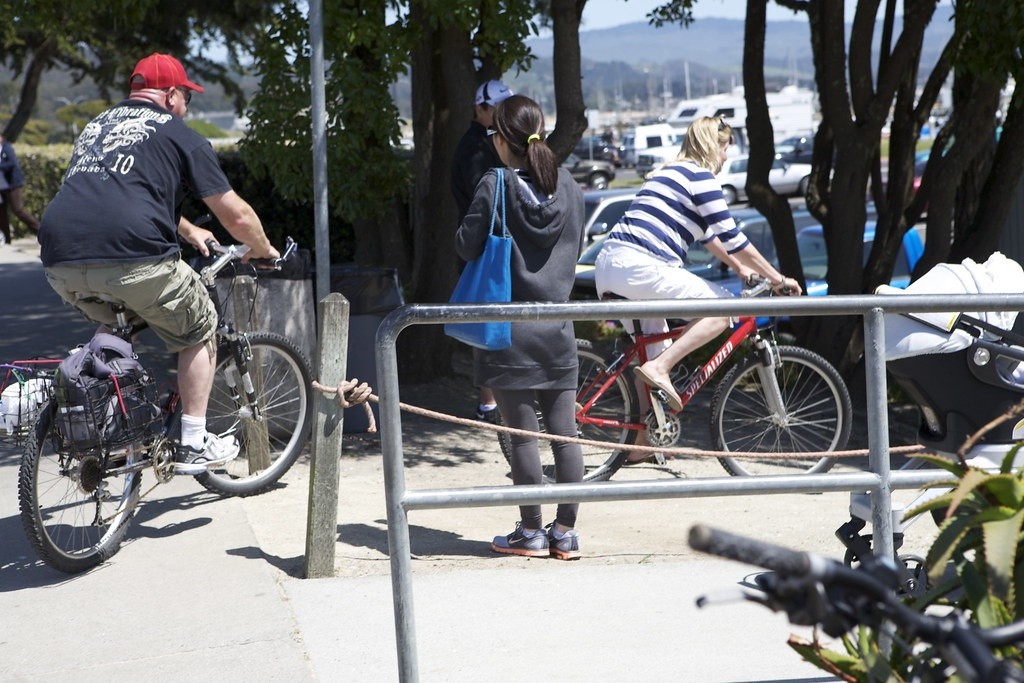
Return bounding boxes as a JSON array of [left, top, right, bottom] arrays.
[[717, 117, 728, 133], [167, 86, 192, 105], [486, 125, 499, 138]]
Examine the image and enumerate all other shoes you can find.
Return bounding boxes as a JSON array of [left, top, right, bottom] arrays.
[[475, 402, 505, 425]]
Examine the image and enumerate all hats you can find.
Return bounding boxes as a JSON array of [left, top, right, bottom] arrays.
[[131, 52, 205, 94], [474, 79, 515, 108]]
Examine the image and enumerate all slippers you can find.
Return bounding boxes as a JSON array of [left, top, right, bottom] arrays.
[[621, 447, 681, 465], [632, 365, 683, 413]]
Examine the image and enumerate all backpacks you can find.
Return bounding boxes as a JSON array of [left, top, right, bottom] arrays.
[[52, 333, 163, 444]]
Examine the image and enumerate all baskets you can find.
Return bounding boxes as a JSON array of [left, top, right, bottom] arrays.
[[46, 367, 165, 456], [0, 355, 64, 449]]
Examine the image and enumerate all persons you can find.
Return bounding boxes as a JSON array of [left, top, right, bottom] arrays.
[[596, 117, 802, 468], [42, 52, 280, 475], [455, 80, 510, 426], [456, 96, 586, 559], [0, 133, 41, 244]]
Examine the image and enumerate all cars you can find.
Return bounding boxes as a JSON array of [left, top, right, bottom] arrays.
[[562, 92, 1004, 298]]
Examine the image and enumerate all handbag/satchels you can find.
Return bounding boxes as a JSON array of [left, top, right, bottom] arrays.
[[444, 168, 511, 351]]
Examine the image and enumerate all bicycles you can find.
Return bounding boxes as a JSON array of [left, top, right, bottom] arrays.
[[686, 288, 1023, 683], [18, 233, 316, 574], [492, 273, 853, 484]]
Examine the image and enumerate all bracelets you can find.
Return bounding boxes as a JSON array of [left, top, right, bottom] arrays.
[[771, 275, 785, 289]]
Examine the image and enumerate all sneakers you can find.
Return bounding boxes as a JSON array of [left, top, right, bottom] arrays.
[[544, 519, 583, 560], [174, 429, 241, 475], [491, 521, 550, 557]]
